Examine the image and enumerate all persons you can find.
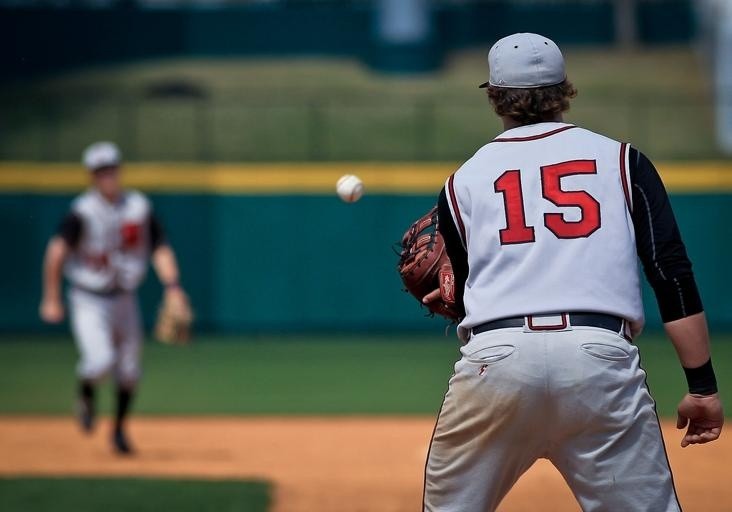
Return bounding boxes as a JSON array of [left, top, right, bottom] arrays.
[[391, 29, 725, 511], [41, 137, 192, 458]]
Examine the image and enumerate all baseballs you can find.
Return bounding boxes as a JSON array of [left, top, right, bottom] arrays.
[[334, 172, 364, 204]]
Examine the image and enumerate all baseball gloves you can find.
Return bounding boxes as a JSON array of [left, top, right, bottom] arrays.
[[400, 205, 464, 321], [154, 301, 193, 350]]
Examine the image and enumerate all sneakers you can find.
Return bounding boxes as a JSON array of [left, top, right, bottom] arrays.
[[113, 429, 131, 454], [80, 404, 94, 430]]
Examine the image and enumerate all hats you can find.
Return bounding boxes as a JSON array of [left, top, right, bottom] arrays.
[[82, 140, 122, 173], [478, 32, 568, 90]]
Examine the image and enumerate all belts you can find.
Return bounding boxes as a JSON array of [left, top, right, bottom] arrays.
[[471, 312, 624, 335]]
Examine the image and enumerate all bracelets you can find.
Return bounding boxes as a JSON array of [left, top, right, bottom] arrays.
[[682, 356, 720, 395]]
